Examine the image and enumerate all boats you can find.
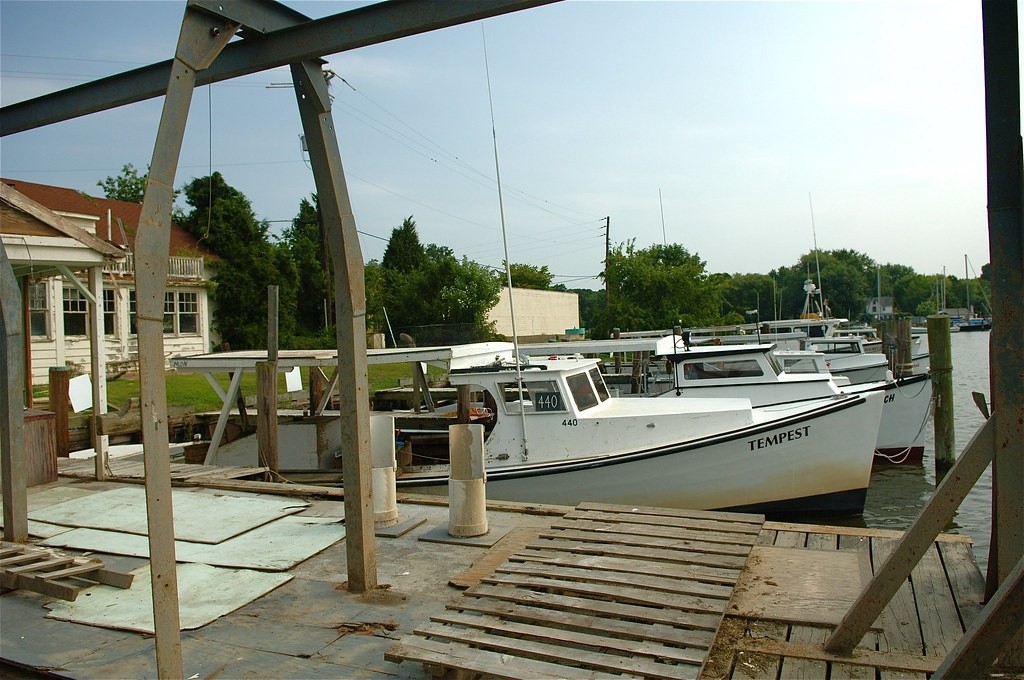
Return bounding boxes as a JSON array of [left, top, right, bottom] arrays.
[[180, 359, 886, 527], [457, 320, 940, 457]]
